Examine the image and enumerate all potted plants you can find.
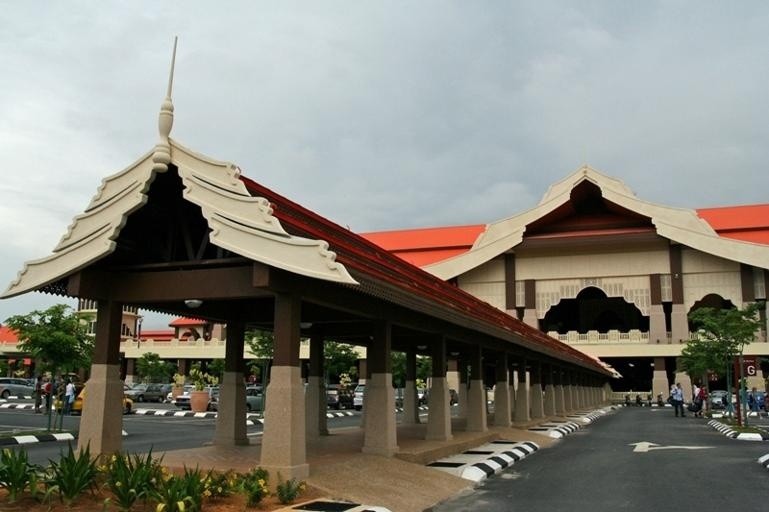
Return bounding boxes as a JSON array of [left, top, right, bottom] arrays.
[[188, 367, 220, 412], [170, 373, 186, 401]]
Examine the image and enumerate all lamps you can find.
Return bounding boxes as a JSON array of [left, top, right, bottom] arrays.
[[184, 299, 203, 308]]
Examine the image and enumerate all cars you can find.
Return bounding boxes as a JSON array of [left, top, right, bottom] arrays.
[[394, 386, 406, 406], [708, 390, 730, 410], [745, 387, 769, 412], [416, 387, 427, 405], [41, 380, 51, 394], [448, 387, 458, 405], [54, 372, 134, 415], [123, 381, 263, 412]]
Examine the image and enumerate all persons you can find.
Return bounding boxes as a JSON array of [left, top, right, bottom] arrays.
[[670, 381, 686, 417], [694, 384, 769, 422], [30, 373, 76, 418]]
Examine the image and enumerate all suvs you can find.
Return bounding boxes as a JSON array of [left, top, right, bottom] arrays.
[[352, 382, 366, 410], [326, 381, 358, 410], [0, 378, 36, 399]]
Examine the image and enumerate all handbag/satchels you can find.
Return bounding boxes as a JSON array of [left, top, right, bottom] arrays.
[[667, 396, 678, 406], [68, 395, 75, 404], [694, 397, 703, 405]]
